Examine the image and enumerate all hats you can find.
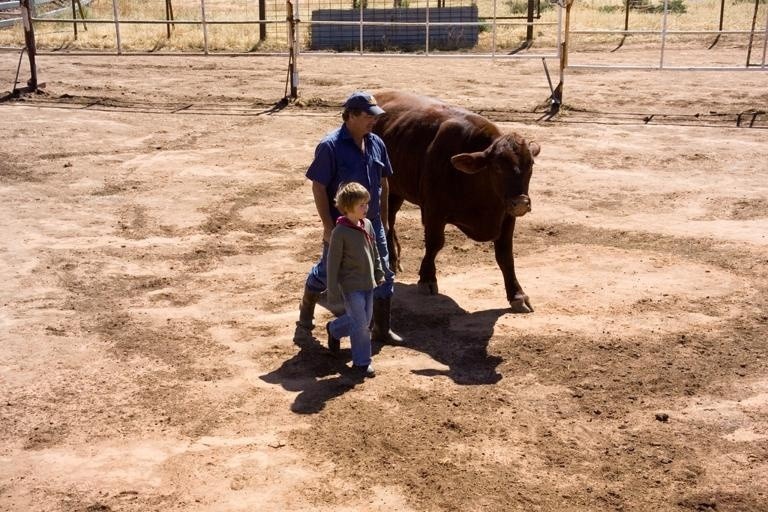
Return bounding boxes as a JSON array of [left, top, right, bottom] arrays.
[[345, 92, 387, 116]]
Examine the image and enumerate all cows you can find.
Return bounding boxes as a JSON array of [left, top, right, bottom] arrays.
[[369, 89, 541, 314]]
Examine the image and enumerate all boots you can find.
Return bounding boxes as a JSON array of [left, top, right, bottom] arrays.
[[297, 285, 321, 330], [372, 297, 404, 343]]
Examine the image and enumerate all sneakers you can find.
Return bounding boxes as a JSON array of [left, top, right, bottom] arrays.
[[351, 364, 376, 378], [326, 321, 341, 352]]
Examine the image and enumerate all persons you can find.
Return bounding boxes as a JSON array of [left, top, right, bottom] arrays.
[[293, 91, 407, 352], [325, 182, 388, 379]]
